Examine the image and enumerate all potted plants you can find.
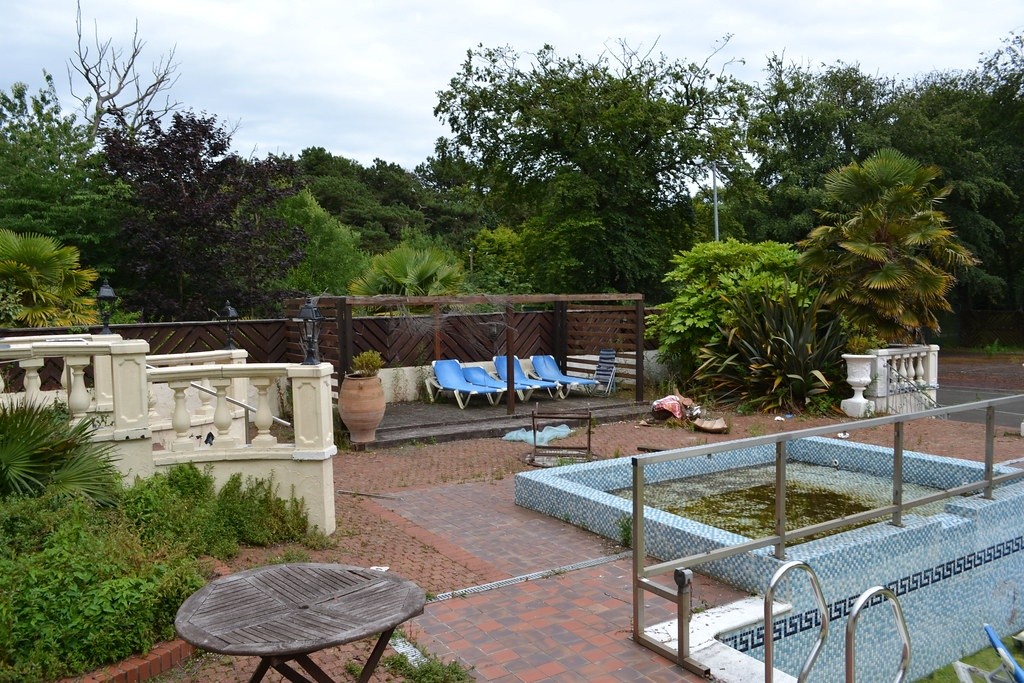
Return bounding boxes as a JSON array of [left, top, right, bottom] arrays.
[[338, 350, 386, 443], [841, 332, 877, 387]]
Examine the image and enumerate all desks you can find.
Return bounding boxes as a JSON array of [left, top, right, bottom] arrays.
[[173, 562, 426, 683]]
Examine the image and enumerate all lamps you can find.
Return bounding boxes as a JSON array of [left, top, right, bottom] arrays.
[[218, 300, 240, 351], [96, 279, 118, 336], [297, 296, 326, 365]]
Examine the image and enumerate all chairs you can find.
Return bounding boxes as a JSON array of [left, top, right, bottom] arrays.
[[528, 354, 600, 399], [461, 367, 532, 407], [591, 349, 616, 397], [425, 359, 503, 410], [488, 355, 563, 403], [953, 622, 1024, 683]]
